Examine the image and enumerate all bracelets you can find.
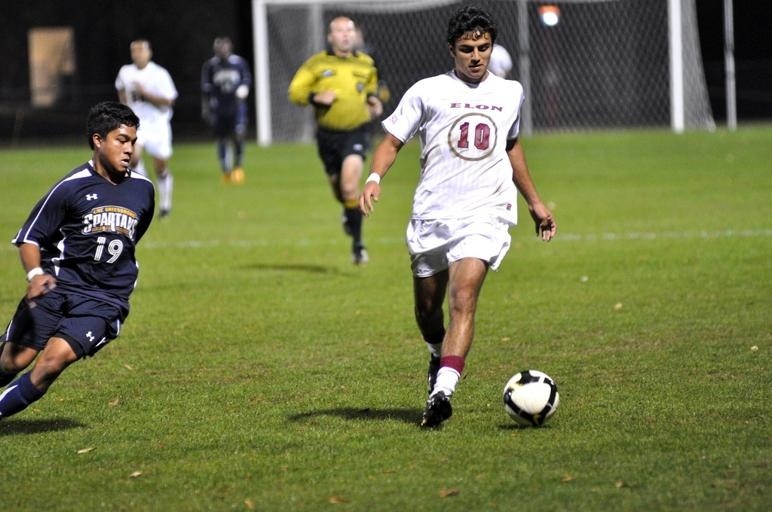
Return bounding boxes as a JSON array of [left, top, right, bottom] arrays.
[[25, 267, 44, 283], [365, 172, 380, 187]]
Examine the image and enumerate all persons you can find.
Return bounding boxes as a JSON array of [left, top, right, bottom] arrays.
[[200, 35, 252, 183], [288, 14, 385, 266], [0, 102, 155, 420], [487, 35, 513, 79], [357, 7, 557, 430], [114, 38, 180, 217]]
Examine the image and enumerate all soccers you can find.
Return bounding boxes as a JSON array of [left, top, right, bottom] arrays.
[[502, 370, 561, 427]]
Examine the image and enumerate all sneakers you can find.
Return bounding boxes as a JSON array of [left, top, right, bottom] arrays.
[[351, 246, 368, 264], [420, 352, 452, 427], [342, 208, 352, 236]]
